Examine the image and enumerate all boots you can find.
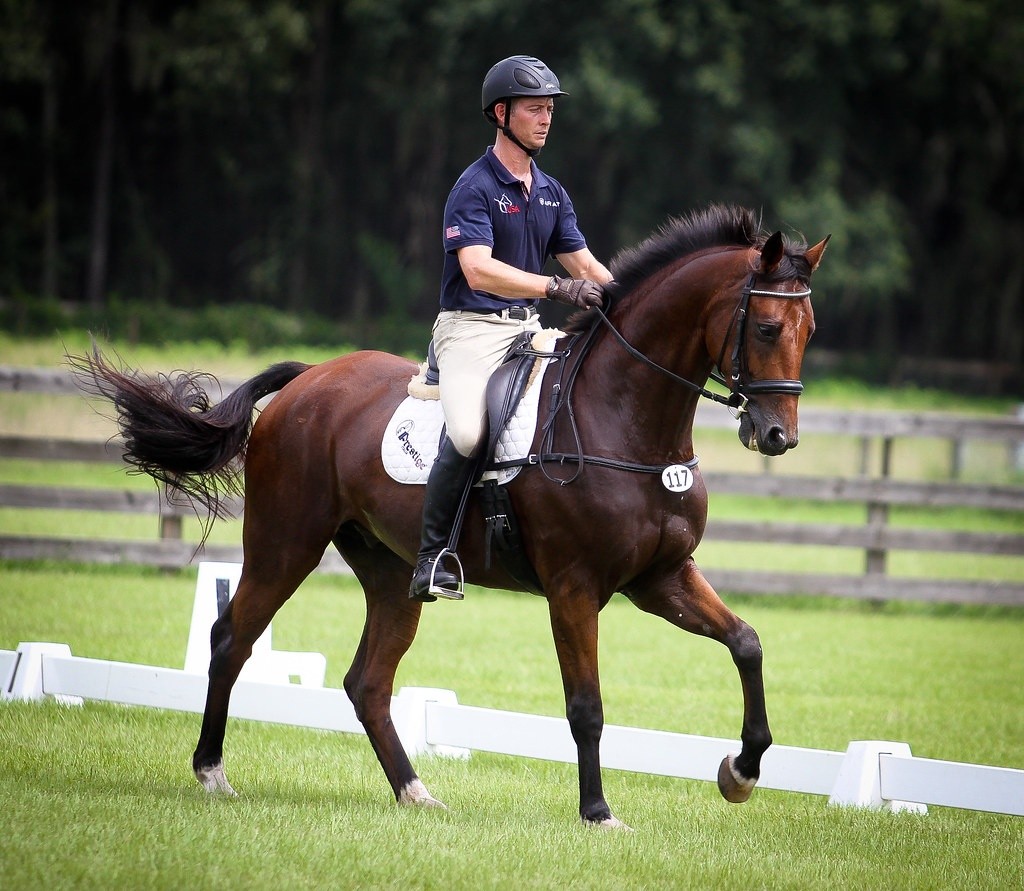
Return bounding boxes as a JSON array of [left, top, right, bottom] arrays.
[[408, 436, 476, 602]]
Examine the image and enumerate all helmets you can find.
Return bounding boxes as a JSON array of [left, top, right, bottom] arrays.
[[481, 54, 570, 126]]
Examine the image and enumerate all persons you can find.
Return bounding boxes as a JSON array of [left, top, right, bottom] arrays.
[[409, 54, 614, 602]]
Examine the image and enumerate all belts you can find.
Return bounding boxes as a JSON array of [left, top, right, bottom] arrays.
[[444, 305, 537, 321]]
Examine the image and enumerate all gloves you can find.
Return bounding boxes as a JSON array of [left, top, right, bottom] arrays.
[[599, 281, 622, 298], [546, 275, 604, 310]]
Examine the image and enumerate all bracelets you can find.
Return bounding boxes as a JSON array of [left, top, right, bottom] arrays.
[[545, 276, 556, 293]]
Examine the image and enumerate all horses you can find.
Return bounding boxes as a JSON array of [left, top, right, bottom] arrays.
[[59, 203, 831, 830]]
